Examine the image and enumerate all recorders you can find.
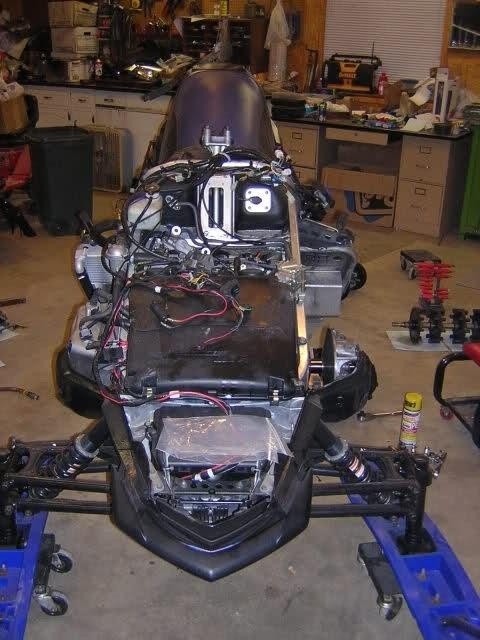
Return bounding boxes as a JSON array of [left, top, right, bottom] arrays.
[[321, 53, 381, 94]]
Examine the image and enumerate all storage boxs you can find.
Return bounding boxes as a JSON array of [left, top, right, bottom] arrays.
[[48, 1, 100, 55], [321, 162, 398, 229], [0, 90, 35, 192]]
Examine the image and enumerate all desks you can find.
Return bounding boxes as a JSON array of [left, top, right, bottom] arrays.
[[266, 114, 476, 240], [15, 60, 176, 181]]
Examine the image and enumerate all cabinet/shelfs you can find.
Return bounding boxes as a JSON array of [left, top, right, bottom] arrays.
[[179, 13, 268, 73]]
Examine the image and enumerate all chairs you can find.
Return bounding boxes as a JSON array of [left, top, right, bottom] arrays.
[[155, 68, 278, 167]]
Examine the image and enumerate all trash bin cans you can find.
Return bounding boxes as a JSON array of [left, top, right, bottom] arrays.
[[27, 126, 93, 236]]
